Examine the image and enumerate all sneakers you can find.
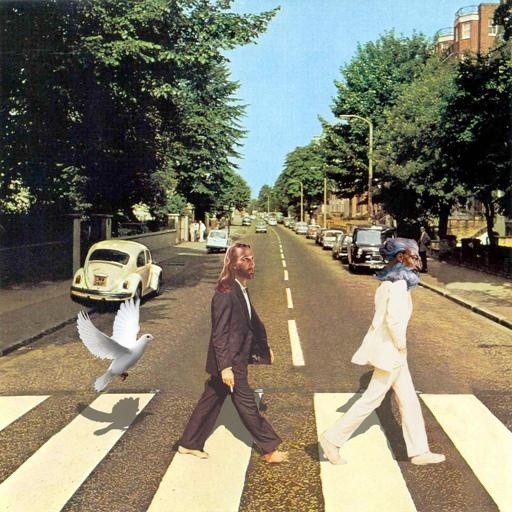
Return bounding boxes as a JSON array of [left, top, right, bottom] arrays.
[[410, 450, 447, 466], [320, 432, 349, 466]]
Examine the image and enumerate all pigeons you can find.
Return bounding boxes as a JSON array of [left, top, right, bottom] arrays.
[[76, 296, 155, 392]]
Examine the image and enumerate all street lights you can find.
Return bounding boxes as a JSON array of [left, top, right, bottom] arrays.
[[339, 113, 375, 220], [289, 180, 303, 221]]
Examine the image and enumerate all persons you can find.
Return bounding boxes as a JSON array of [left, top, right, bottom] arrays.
[[416, 226, 432, 274], [197, 220, 207, 241], [175, 242, 293, 465], [317, 236, 446, 466], [193, 219, 199, 242]]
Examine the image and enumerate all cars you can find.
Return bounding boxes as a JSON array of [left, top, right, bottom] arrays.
[[283, 216, 399, 274], [243, 210, 284, 234], [69, 239, 164, 309]]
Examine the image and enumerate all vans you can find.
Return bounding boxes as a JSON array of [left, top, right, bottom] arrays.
[[206, 230, 230, 252]]
